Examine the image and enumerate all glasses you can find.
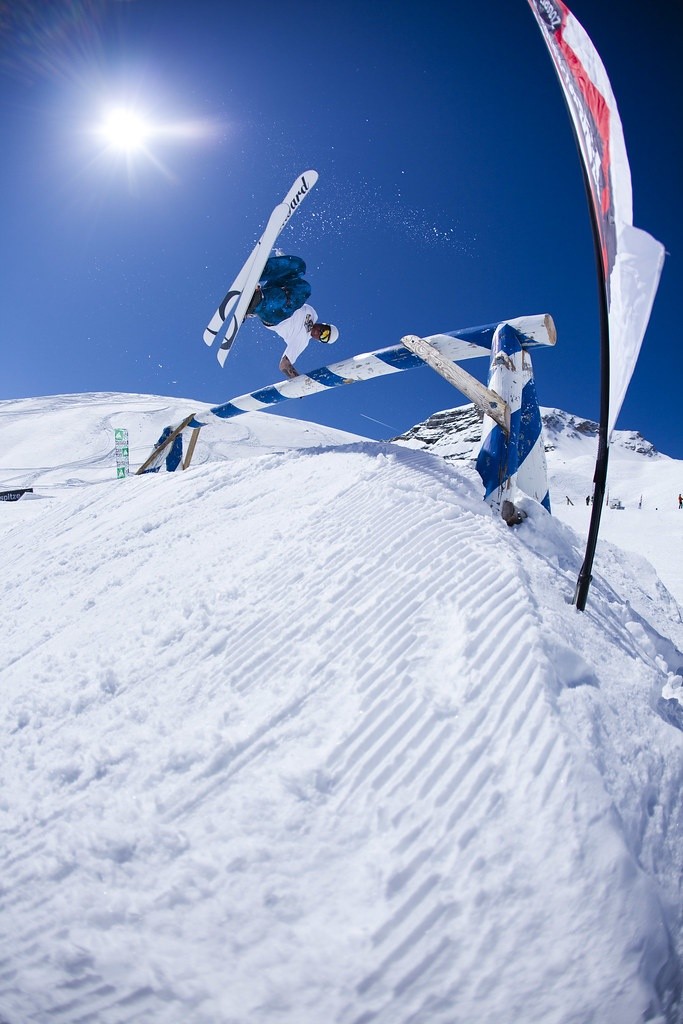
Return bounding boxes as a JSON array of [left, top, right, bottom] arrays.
[[319, 325, 330, 343]]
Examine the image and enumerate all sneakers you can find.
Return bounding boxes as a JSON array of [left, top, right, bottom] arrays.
[[245, 290, 264, 320]]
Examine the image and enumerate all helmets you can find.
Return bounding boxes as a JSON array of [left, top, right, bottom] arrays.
[[321, 322, 340, 344]]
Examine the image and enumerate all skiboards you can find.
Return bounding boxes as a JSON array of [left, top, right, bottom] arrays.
[[202, 170, 319, 368]]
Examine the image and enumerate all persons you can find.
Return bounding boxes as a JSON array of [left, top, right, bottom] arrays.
[[243, 255, 339, 400], [678, 494, 683, 509], [586, 495, 590, 505]]
